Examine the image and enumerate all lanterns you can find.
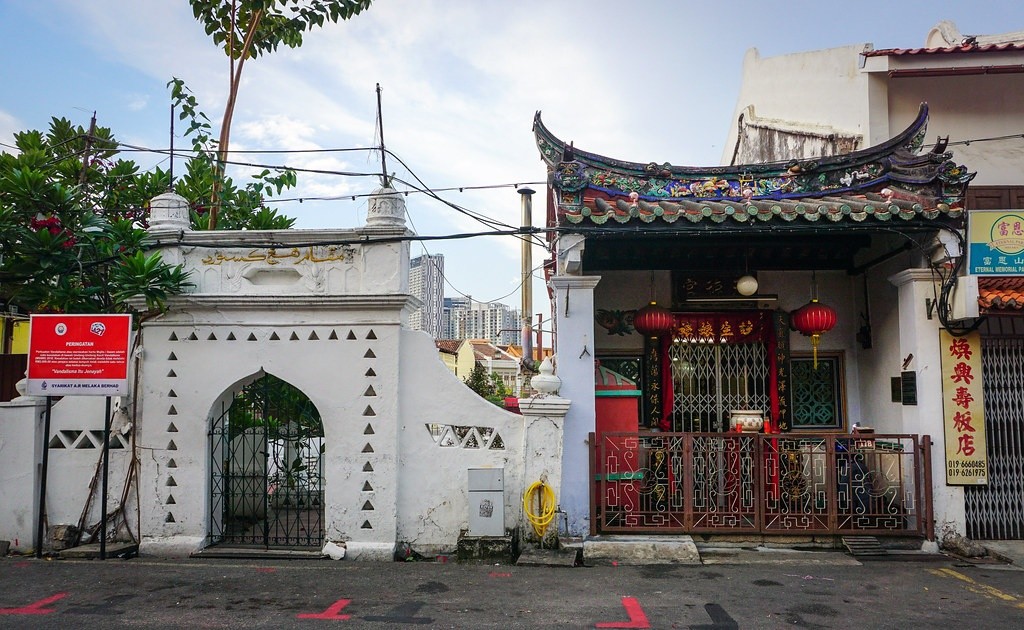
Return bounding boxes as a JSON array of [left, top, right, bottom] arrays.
[[634, 301, 674, 342], [793, 299, 838, 370]]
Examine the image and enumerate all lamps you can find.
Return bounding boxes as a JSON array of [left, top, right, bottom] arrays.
[[737, 248, 758, 296]]
